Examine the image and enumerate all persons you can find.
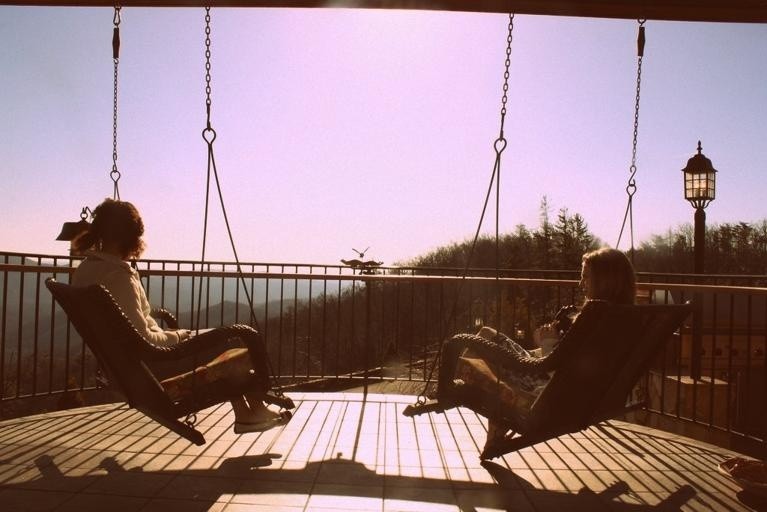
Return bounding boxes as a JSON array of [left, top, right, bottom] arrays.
[[71, 199, 295, 433], [427, 242, 639, 412]]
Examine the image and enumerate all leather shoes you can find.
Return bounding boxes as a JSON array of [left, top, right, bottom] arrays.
[[234, 417, 283, 434], [275, 411, 292, 426]]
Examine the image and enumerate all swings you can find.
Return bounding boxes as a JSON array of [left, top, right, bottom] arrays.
[[401, 12, 691, 460], [43, 5, 294, 445]]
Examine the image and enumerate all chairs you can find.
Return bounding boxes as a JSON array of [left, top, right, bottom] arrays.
[[42, 271, 295, 447], [397, 295, 696, 469]]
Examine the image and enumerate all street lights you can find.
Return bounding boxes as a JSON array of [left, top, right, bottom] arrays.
[[678, 137, 718, 381]]
